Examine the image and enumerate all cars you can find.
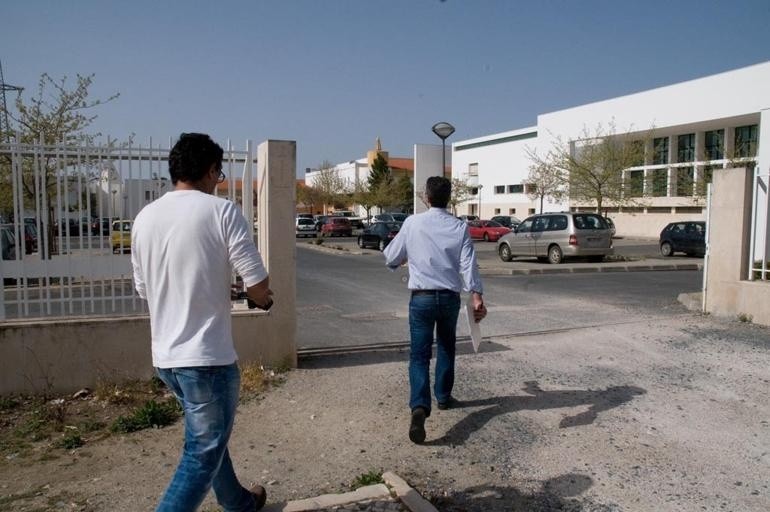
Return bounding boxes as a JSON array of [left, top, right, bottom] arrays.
[[56, 216, 122, 235], [0, 215, 55, 259], [296, 211, 409, 252], [458, 215, 526, 242]]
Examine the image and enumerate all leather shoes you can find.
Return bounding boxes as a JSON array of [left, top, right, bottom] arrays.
[[240, 483, 267, 511], [436, 396, 460, 411]]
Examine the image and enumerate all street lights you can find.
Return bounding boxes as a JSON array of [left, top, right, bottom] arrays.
[[432, 122, 455, 178]]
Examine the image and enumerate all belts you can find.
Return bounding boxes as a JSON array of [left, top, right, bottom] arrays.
[[408, 405, 427, 444], [412, 288, 453, 297]]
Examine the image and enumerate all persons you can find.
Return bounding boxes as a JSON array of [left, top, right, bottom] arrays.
[[129, 133, 275, 512], [381, 177, 487, 442]]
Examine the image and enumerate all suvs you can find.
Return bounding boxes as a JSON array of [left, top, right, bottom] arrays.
[[108, 220, 134, 252]]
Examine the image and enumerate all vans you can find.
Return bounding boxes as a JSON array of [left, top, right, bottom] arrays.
[[497, 211, 615, 264], [660, 222, 706, 258]]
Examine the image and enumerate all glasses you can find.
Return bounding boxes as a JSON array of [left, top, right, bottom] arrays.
[[218, 167, 228, 185]]
